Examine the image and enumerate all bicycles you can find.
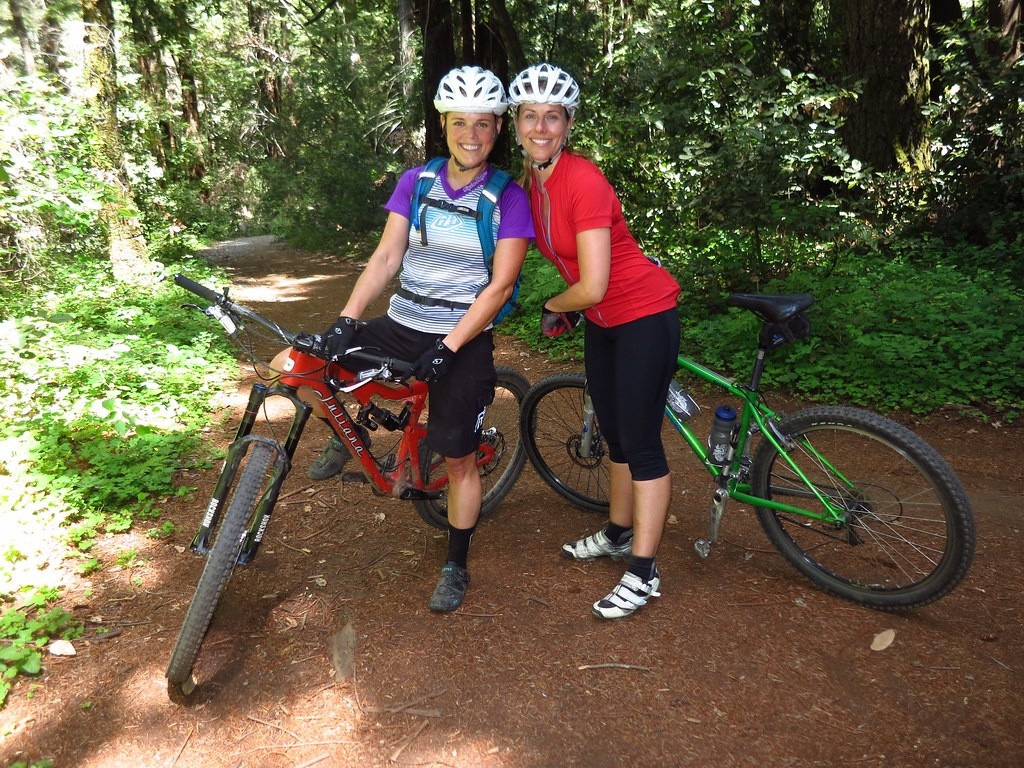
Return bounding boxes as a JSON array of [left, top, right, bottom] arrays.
[[519, 256, 977, 612], [164, 273, 535, 684]]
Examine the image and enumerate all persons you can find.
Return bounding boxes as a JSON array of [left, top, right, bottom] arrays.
[[505, 62, 684, 621], [270, 66, 534, 613]]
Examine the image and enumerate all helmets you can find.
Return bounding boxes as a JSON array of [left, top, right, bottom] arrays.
[[432, 66, 506, 116], [508, 60, 581, 113]]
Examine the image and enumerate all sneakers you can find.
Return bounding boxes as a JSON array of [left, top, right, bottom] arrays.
[[560, 529, 635, 559], [429, 562, 471, 614], [306, 429, 373, 480], [589, 572, 664, 620]]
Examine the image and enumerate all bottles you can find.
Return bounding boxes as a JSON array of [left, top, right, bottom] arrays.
[[665, 380, 699, 423], [706, 404, 737, 465]]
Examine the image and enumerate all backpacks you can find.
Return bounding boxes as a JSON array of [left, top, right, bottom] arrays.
[[405, 155, 522, 323]]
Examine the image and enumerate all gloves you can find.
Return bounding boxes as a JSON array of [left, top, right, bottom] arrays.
[[540, 299, 578, 339], [398, 340, 456, 385], [318, 315, 359, 364]]
